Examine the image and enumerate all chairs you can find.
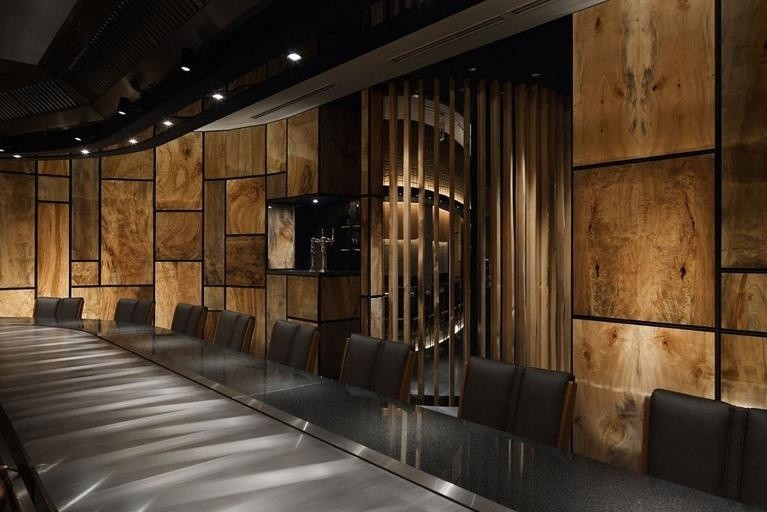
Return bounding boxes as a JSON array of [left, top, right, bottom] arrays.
[[211, 310, 255, 354], [113, 298, 155, 325], [338, 333, 416, 403], [457, 356, 577, 450], [641, 388, 767, 511], [34, 297, 84, 320], [171, 303, 208, 339], [268, 320, 321, 373]]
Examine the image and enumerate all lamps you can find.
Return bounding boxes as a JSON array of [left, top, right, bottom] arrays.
[[117, 96, 129, 116], [180, 50, 194, 72]]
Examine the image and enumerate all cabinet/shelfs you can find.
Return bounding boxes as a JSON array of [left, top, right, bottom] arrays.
[[268, 269, 361, 376]]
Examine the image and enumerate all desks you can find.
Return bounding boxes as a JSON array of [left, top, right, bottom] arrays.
[[0, 317, 767, 511]]
